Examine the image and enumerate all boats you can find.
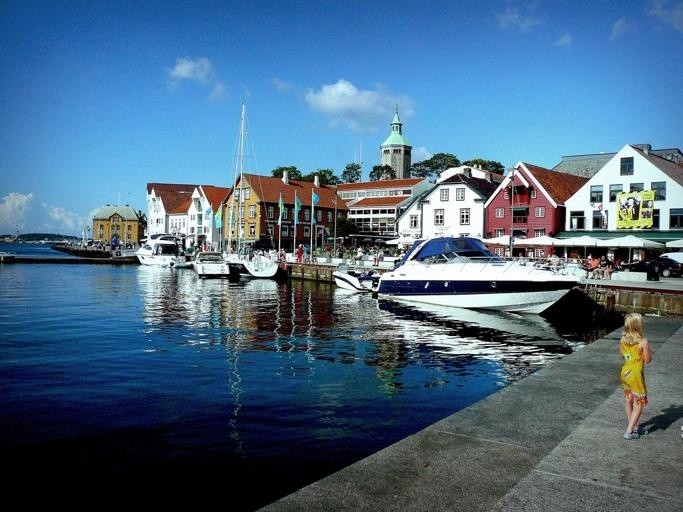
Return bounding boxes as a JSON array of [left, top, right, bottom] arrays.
[[193, 249, 229, 279], [378, 236, 582, 316], [332, 268, 381, 294], [135, 239, 193, 269]]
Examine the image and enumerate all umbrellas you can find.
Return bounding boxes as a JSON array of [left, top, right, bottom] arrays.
[[593, 234, 665, 265], [515, 235, 560, 257], [481, 235, 522, 258], [384, 235, 426, 245], [665, 239, 682, 248], [553, 235, 604, 259]]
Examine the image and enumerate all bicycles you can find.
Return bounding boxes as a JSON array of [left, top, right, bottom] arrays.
[[304, 255, 318, 265]]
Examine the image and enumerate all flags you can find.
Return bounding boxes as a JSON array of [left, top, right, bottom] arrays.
[[310, 187, 319, 225], [330, 183, 337, 207], [228, 200, 235, 233], [276, 191, 285, 227], [214, 201, 222, 229], [204, 202, 212, 230], [293, 189, 303, 226]]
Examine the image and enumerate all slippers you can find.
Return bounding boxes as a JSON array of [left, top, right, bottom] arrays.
[[634, 427, 649, 434], [623, 432, 640, 440]]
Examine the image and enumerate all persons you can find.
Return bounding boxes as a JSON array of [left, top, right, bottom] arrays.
[[618, 310, 651, 441], [64, 235, 145, 251], [619, 197, 637, 222], [642, 200, 652, 208], [230, 238, 408, 270], [517, 250, 651, 280]]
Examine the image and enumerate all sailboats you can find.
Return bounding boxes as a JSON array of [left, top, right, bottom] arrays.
[[224, 100, 292, 282]]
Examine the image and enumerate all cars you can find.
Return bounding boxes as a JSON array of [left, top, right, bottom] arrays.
[[621, 257, 682, 277]]
